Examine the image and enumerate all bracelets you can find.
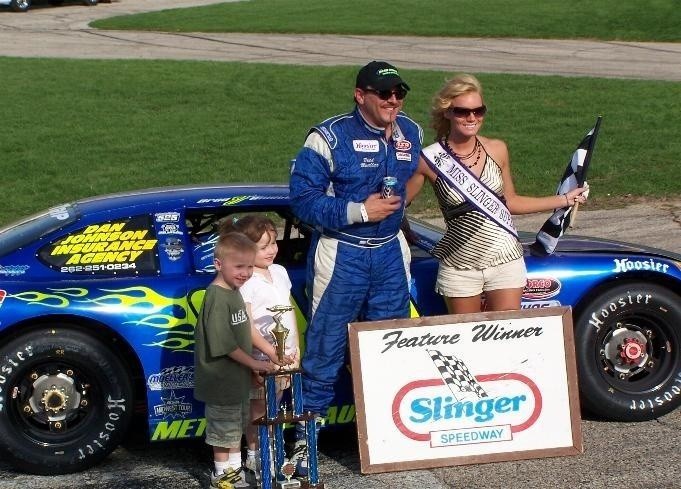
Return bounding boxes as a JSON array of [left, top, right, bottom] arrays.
[[565, 192, 571, 211], [360, 203, 370, 223]]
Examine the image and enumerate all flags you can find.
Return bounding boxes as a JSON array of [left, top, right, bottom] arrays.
[[429, 349, 488, 400], [530, 114, 602, 260]]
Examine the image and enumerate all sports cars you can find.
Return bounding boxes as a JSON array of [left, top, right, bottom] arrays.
[[1, 183, 681, 473]]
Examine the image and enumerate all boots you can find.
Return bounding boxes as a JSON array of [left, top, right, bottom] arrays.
[[287, 415, 325, 476]]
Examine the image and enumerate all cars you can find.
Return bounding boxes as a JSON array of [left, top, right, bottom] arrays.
[[1, 0, 112, 13]]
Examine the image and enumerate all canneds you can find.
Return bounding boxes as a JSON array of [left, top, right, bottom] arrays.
[[380, 177, 397, 199]]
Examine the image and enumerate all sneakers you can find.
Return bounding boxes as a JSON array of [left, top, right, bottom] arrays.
[[208, 466, 256, 489], [245, 443, 276, 480]]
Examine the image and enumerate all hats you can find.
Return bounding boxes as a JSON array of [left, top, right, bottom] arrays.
[[355, 60, 411, 92]]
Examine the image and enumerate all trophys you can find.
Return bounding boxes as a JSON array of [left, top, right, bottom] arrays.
[[252, 305, 327, 489]]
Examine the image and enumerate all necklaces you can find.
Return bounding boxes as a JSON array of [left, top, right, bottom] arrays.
[[444, 130, 484, 170]]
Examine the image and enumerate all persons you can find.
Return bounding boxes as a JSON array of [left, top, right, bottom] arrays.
[[220, 216, 305, 479], [288, 61, 428, 480], [192, 232, 277, 489], [403, 72, 589, 313]]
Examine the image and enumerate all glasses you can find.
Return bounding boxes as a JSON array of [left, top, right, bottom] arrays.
[[362, 86, 408, 101], [449, 104, 488, 117]]
